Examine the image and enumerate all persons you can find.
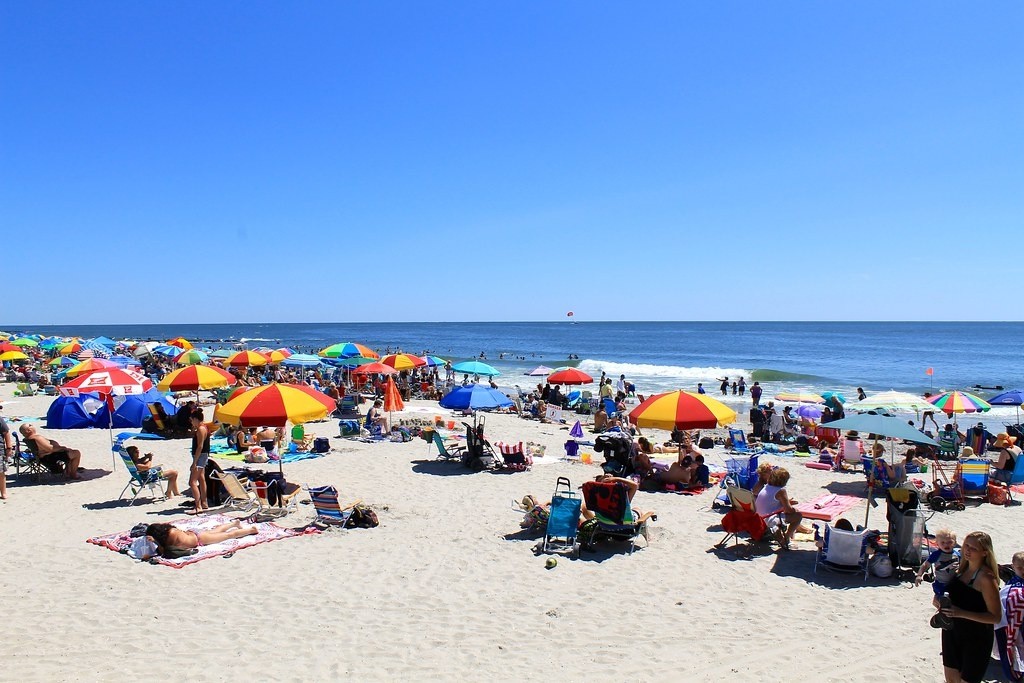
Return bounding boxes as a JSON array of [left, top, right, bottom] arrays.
[[0, 346, 579, 399], [523, 370, 1024, 575], [147, 518, 259, 551], [915, 529, 1024, 683], [0, 400, 396, 514]]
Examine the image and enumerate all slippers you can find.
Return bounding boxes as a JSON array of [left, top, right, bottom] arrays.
[[179, 500, 195, 507], [777, 539, 787, 551], [930, 594, 953, 631], [223, 550, 234, 558]]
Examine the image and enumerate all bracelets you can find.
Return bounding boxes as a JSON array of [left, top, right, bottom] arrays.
[[7, 447, 12, 449]]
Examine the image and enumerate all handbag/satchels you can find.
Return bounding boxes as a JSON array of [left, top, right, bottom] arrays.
[[986, 480, 1011, 505], [868, 552, 892, 578], [933, 478, 961, 500]]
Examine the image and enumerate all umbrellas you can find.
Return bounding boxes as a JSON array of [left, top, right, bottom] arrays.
[[524, 364, 551, 388], [820, 391, 846, 407], [383, 374, 405, 427], [547, 369, 593, 408], [987, 389, 1024, 424], [774, 388, 826, 430], [225, 385, 258, 401], [926, 389, 991, 451], [844, 390, 941, 464], [627, 389, 736, 464], [214, 380, 337, 472], [155, 363, 236, 406], [57, 368, 152, 470], [451, 359, 500, 382], [0, 329, 448, 376], [548, 365, 578, 395], [795, 404, 823, 418], [821, 413, 940, 526], [438, 381, 515, 446]]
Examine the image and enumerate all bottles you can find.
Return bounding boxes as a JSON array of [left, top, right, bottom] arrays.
[[941, 592, 951, 609]]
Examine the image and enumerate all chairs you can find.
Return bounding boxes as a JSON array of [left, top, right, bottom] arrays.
[[333, 396, 358, 419], [577, 398, 1024, 582], [11, 431, 69, 484], [432, 431, 467, 465], [258, 424, 317, 453], [302, 483, 363, 532], [9, 370, 40, 395], [209, 468, 301, 518], [146, 402, 191, 438], [43, 385, 61, 395], [413, 382, 429, 400], [495, 443, 534, 471], [112, 440, 172, 507], [515, 385, 533, 420]]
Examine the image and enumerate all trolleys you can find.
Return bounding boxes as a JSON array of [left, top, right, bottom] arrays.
[[926, 478, 965, 511]]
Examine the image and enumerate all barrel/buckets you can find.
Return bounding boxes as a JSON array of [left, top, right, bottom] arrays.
[[581, 454, 590, 463], [435, 416, 441, 424], [448, 420, 455, 429]]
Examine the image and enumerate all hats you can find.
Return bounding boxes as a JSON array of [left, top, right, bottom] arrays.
[[956, 447, 978, 461], [611, 412, 618, 418], [681, 456, 692, 468], [784, 406, 792, 413], [993, 433, 1017, 448]]
